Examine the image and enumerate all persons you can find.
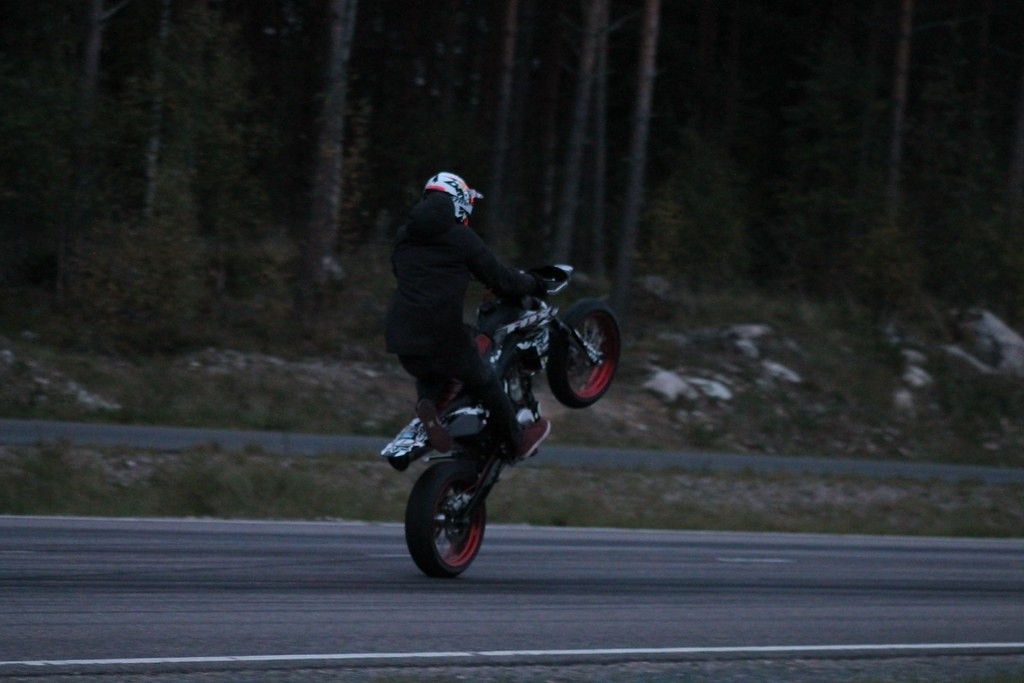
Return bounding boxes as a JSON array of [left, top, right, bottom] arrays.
[[385, 171, 551, 461]]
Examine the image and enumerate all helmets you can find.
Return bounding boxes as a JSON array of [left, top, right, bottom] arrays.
[[422, 172, 482, 226]]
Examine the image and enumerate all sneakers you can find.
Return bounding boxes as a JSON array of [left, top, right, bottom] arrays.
[[515, 419, 551, 461], [415, 399, 451, 454]]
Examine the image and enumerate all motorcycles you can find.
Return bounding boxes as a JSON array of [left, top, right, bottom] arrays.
[[379, 264, 622, 579]]
